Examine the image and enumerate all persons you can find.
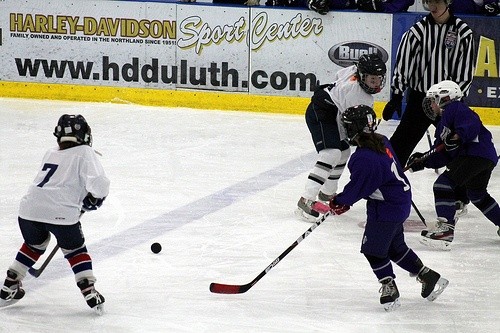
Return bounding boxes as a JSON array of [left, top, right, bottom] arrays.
[[179, 0, 500, 18], [383, 0, 475, 174], [0, 115, 109, 315], [297, 54, 388, 222], [406, 79, 500, 250], [328, 105, 448, 311]]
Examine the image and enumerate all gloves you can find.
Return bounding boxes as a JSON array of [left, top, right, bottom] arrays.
[[329, 195, 350, 216], [83, 192, 106, 210], [407, 152, 424, 171], [382, 94, 403, 121]]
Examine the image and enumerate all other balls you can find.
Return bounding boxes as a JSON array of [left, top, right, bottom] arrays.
[[151, 243, 162, 254]]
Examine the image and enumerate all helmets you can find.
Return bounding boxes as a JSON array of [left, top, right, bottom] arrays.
[[53, 113, 92, 146], [357, 54, 386, 94], [307, 0, 329, 15], [422, 80, 462, 122], [342, 104, 377, 146], [422, 0, 451, 11]]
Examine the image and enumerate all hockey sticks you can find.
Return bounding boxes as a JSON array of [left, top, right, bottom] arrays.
[[208, 206, 332, 295], [410, 200, 429, 227], [427, 126, 468, 217], [310, 166, 410, 215], [27, 207, 87, 278]]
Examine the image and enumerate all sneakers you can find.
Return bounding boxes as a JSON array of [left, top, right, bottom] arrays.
[[378, 276, 401, 312], [296, 198, 338, 222], [416, 265, 449, 301], [0, 268, 25, 306], [419, 217, 455, 250], [454, 201, 468, 216], [77, 278, 105, 315]]
